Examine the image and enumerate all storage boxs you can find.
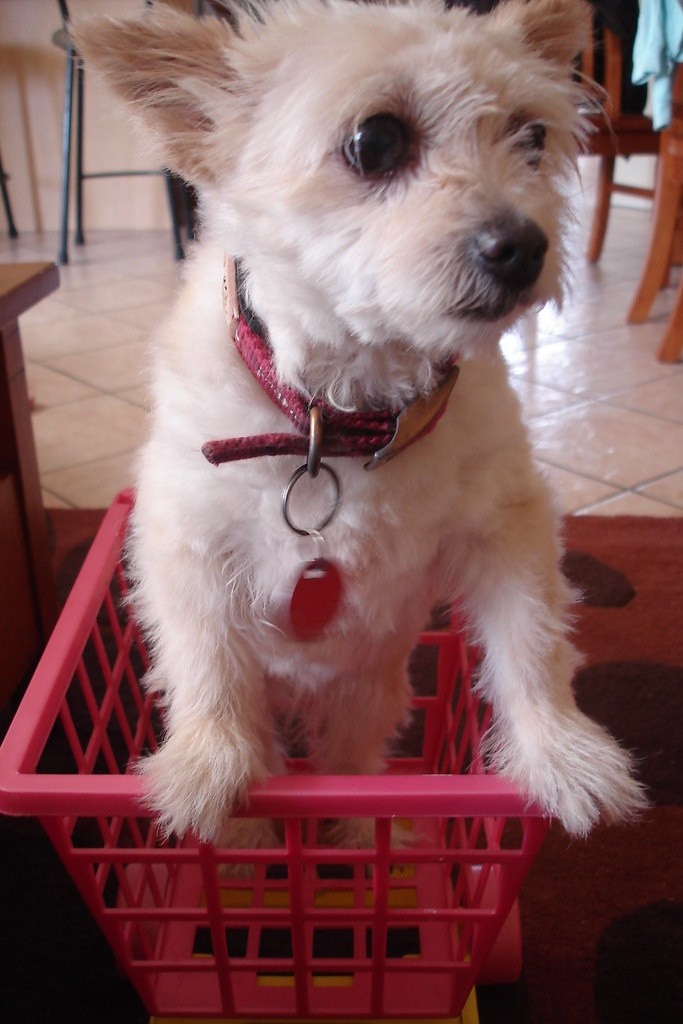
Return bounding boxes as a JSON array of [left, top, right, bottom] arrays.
[[1, 486, 554, 1024]]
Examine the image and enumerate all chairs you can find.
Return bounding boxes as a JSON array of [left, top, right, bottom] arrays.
[[632, 0, 683, 365], [572, 0, 674, 291]]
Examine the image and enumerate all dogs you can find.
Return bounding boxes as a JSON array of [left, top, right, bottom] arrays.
[[63, 0, 654, 841]]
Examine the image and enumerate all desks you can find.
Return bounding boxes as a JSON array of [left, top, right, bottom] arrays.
[[0, 262, 60, 749]]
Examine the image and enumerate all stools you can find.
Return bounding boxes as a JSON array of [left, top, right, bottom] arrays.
[[47, 0, 188, 265]]
